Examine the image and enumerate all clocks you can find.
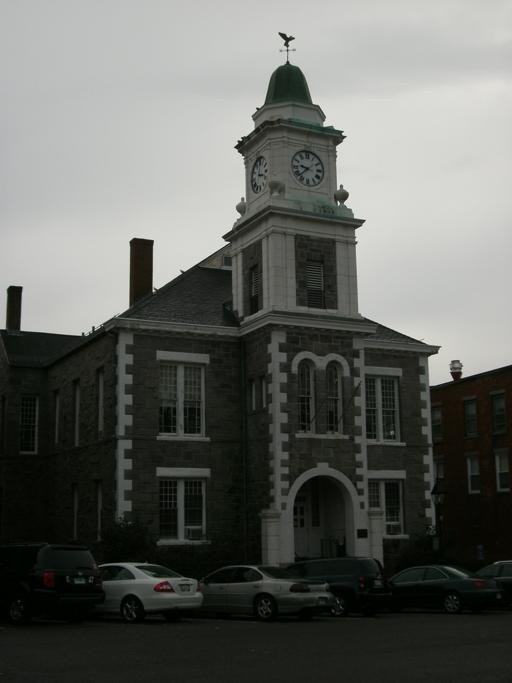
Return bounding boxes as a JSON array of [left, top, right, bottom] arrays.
[[251, 155, 269, 194], [291, 150, 325, 187]]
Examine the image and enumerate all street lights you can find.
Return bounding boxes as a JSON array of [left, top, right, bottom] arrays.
[[431, 478, 449, 558]]
